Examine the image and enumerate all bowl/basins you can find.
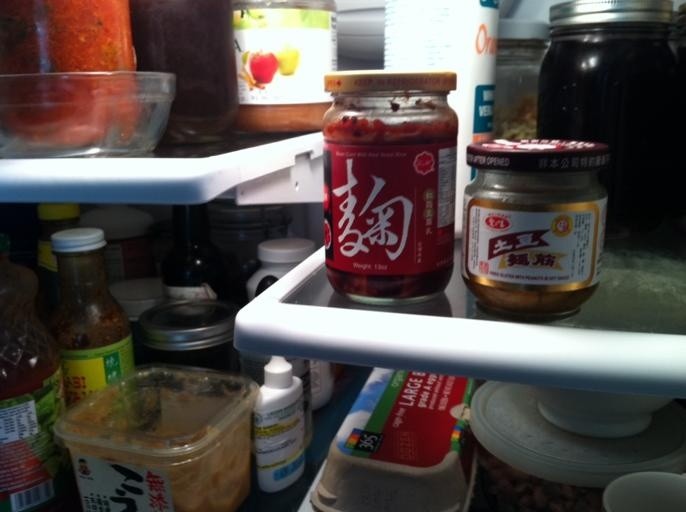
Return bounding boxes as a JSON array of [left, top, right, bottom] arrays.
[[0, 69, 178, 162]]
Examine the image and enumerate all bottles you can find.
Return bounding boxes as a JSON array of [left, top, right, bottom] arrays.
[[0, 202, 332, 512]]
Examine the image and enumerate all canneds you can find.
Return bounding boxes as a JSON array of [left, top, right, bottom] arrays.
[[537, 0, 677, 232], [316, 68, 458, 305], [462, 137, 610, 319], [491, 17, 549, 141], [0, 0, 337, 146]]
